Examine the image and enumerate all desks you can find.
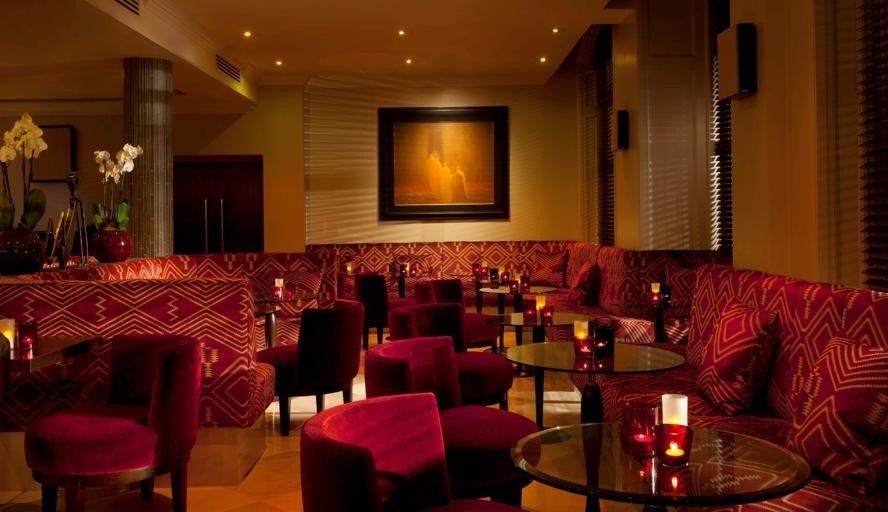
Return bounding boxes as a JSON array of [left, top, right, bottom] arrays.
[[1, 334, 103, 431], [511, 420, 813, 512], [504, 340, 686, 424]]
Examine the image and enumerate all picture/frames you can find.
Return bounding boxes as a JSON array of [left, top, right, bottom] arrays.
[[377, 105, 510, 220]]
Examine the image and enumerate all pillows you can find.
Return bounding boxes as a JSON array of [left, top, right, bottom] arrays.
[[785, 335, 887, 506], [697, 296, 781, 417]]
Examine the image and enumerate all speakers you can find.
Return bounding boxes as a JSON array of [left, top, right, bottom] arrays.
[[717, 23, 756, 101], [610, 110, 629, 152]]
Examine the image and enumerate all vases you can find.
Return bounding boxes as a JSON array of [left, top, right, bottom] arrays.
[[0, 229, 44, 275], [89, 230, 133, 263]]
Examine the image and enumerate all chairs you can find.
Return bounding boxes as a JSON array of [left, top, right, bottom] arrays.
[[387, 301, 514, 412], [364, 336, 541, 508], [298, 390, 532, 511], [22, 333, 203, 511], [257, 297, 363, 437]]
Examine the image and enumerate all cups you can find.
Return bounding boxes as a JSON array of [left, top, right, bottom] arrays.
[[397, 260, 418, 276], [622, 400, 660, 455], [662, 394, 689, 427], [17, 323, 37, 350], [573, 320, 614, 358], [624, 457, 695, 497], [0, 318, 16, 350], [573, 357, 615, 372], [649, 282, 673, 307], [270, 277, 298, 302], [9, 350, 34, 360], [652, 425, 693, 466], [522, 296, 554, 321], [470, 261, 529, 292]]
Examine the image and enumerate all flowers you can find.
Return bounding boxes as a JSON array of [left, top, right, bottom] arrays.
[[0, 110, 48, 231], [84, 135, 144, 233]]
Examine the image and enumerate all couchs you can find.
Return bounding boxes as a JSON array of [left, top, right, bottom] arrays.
[[2, 239, 887, 353], [1, 279, 277, 427], [569, 264, 888, 511]]
